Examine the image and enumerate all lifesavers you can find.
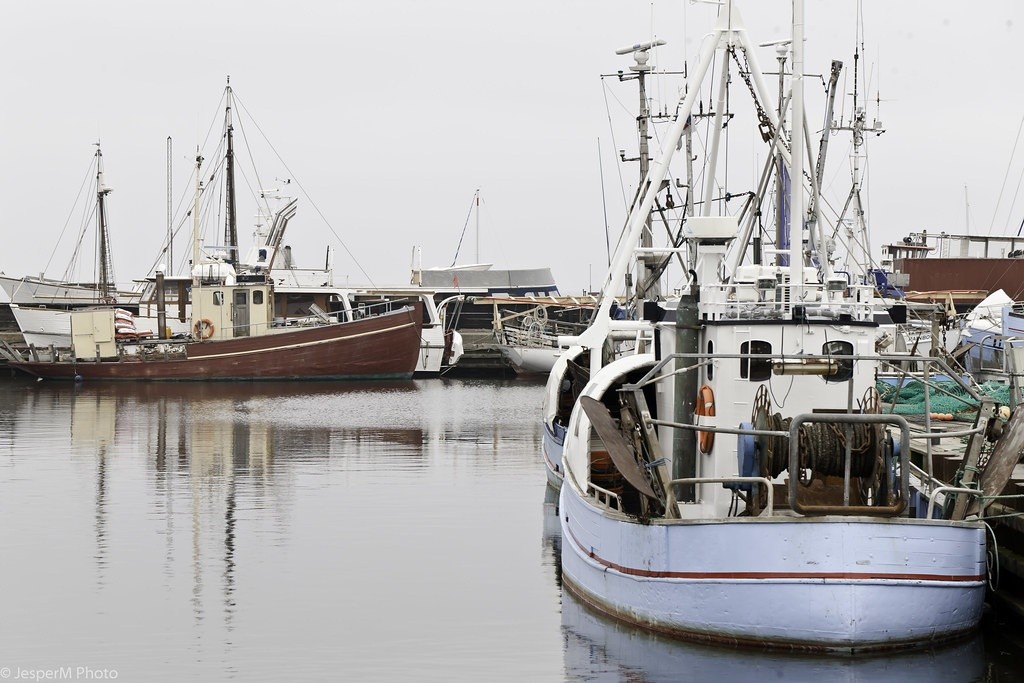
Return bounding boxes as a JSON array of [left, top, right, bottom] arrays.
[[195, 319, 214, 338], [697, 386, 715, 454]]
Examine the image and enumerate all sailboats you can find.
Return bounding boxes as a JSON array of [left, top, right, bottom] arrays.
[[2, 0, 1022, 660]]
